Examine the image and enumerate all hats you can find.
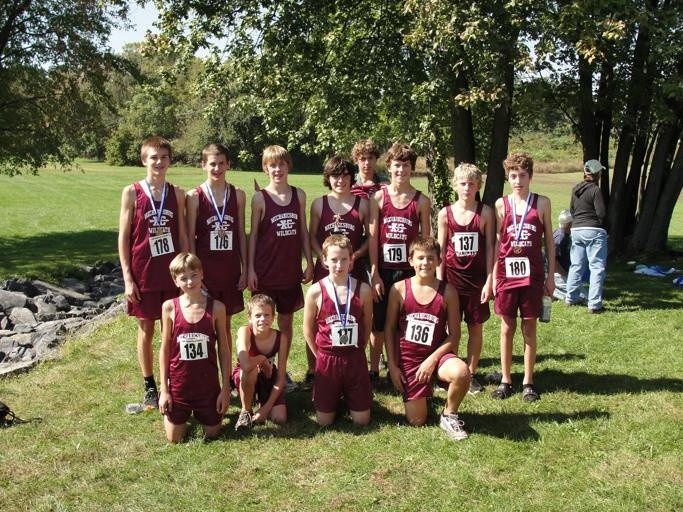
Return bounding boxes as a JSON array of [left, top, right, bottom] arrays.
[[559, 209, 573, 223], [584, 159, 606, 175]]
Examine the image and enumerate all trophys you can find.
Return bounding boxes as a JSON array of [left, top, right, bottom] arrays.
[[327, 213, 348, 236]]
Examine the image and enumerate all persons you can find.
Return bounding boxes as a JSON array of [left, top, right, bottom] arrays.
[[352, 140, 388, 278], [117, 136, 186, 411], [306, 234, 374, 427], [368, 143, 430, 383], [158, 253, 230, 444], [544, 209, 589, 303], [234, 294, 289, 430], [565, 158, 609, 304], [187, 143, 247, 400], [385, 235, 470, 443], [303, 157, 368, 392], [248, 144, 314, 395], [436, 162, 495, 393], [493, 154, 556, 402]]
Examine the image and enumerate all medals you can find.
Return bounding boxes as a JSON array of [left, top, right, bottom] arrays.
[[513, 247, 522, 254], [218, 228, 226, 238], [155, 230, 163, 236], [339, 334, 349, 344]]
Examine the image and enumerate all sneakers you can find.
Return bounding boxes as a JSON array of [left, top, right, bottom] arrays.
[[144, 388, 159, 409], [522, 384, 541, 404], [285, 372, 300, 395], [468, 373, 484, 394], [234, 408, 253, 433], [369, 370, 379, 382], [229, 375, 240, 399], [438, 409, 468, 440], [301, 368, 315, 392], [491, 383, 513, 400]]
